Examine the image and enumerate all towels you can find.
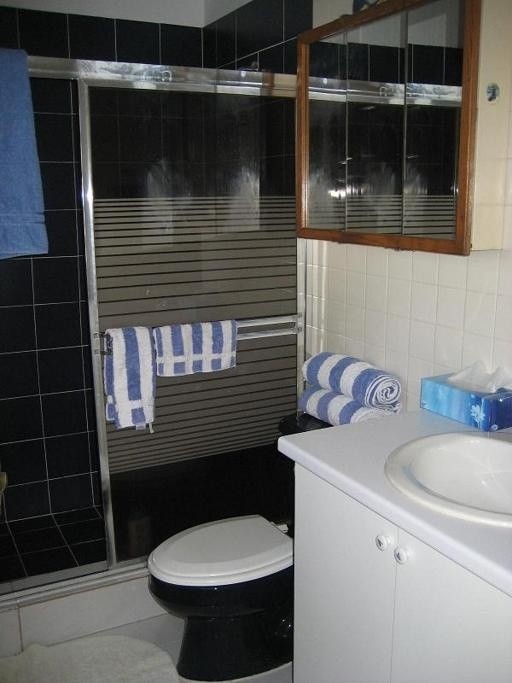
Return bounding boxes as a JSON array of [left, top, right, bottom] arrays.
[[0, 48, 49, 261], [300, 351, 401, 429], [102, 317, 238, 432]]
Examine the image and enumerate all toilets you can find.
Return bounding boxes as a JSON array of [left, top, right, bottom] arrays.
[[147, 412, 334, 681]]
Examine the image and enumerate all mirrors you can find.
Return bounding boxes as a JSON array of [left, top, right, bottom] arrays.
[[295, 1, 473, 256]]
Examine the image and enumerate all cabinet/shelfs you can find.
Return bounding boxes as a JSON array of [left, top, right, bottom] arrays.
[[291, 464, 511, 683]]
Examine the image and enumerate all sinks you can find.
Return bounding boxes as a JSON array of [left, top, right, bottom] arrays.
[[385, 429, 512, 528]]
